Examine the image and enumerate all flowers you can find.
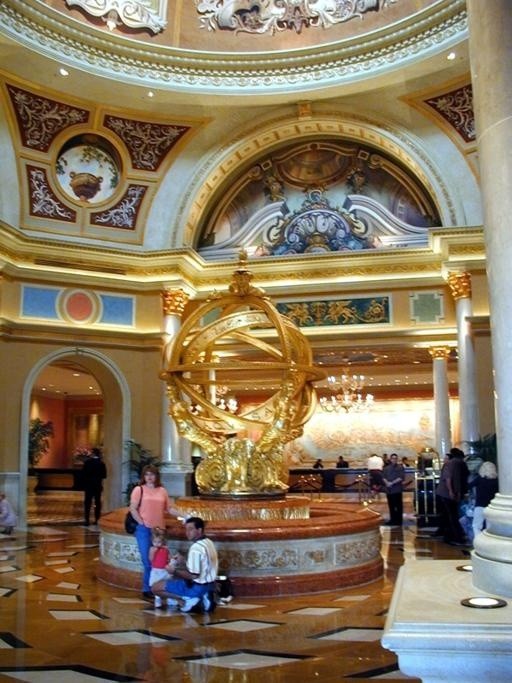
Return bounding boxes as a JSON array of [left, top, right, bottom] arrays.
[[71, 447, 93, 458]]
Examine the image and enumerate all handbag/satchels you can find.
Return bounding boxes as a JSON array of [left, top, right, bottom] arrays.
[[125, 512, 138, 534]]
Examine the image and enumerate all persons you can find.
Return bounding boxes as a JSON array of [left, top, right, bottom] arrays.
[[313, 459, 324, 467], [151, 517, 219, 612], [429, 447, 497, 546], [130, 465, 186, 599], [368, 451, 411, 499], [0, 492, 17, 534], [149, 529, 178, 608], [336, 455, 349, 468], [79, 449, 107, 526], [382, 453, 405, 526]]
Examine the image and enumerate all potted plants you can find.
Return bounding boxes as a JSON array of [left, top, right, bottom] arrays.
[[28, 417, 55, 497]]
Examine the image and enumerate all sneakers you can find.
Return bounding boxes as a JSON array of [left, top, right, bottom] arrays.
[[203, 590, 215, 611], [144, 591, 155, 598], [154, 595, 162, 608], [166, 597, 179, 606], [180, 597, 201, 612]]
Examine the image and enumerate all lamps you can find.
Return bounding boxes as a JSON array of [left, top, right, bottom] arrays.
[[187, 384, 239, 416], [319, 365, 378, 417]]
